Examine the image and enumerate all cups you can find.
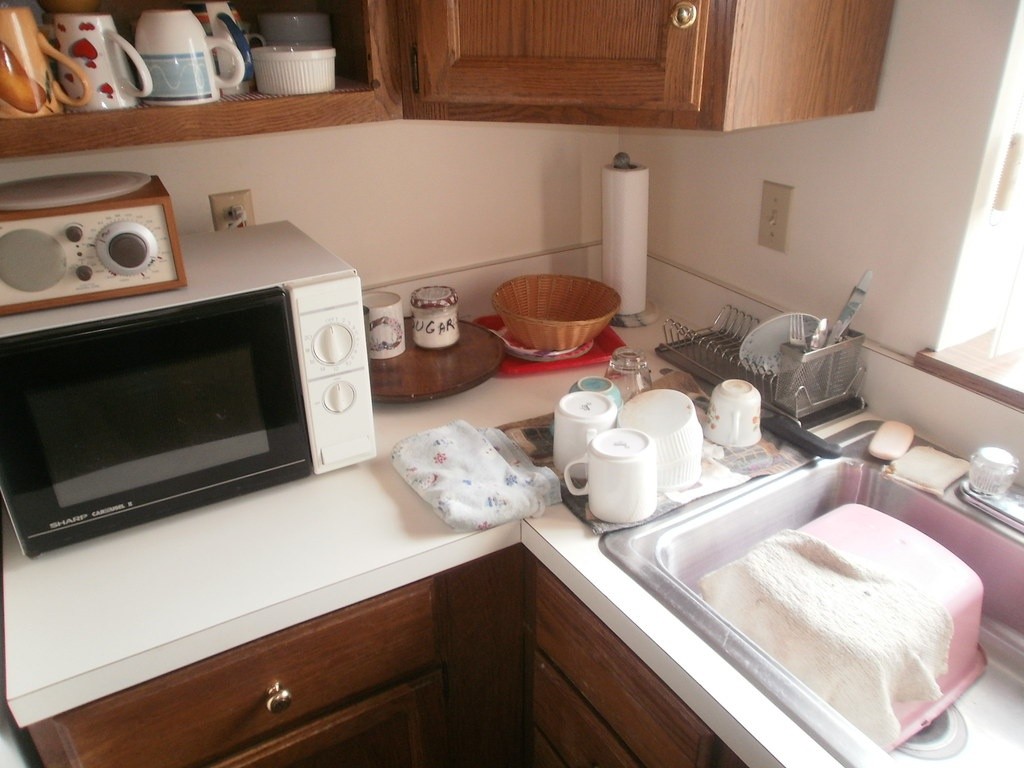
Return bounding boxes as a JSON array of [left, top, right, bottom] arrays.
[[569, 376, 623, 428], [553, 391, 618, 478], [38, 13, 153, 110], [703, 379, 762, 447], [616, 388, 704, 489], [564, 428, 657, 522], [362, 291, 406, 359], [135, 0, 267, 106], [0, 7, 93, 118]]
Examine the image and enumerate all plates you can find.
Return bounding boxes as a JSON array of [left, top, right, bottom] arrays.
[[739, 313, 821, 374]]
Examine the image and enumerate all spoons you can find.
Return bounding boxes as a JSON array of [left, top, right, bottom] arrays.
[[811, 318, 828, 350]]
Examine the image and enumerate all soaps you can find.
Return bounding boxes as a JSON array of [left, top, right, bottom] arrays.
[[866, 420, 915, 460]]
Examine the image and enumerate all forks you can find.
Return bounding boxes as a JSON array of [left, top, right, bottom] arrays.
[[789, 313, 809, 350]]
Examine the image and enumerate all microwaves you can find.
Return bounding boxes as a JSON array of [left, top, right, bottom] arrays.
[[0, 220, 377, 559]]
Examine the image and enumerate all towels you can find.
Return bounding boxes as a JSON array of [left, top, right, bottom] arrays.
[[389, 420, 563, 532]]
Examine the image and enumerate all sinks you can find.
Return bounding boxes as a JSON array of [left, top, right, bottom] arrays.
[[652, 462, 1023, 768]]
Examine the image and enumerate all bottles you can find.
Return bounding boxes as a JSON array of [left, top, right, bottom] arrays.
[[258, 13, 332, 47], [411, 286, 460, 348], [605, 346, 652, 405]]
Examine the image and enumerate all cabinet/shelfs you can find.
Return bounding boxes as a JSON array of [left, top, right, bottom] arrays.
[[523, 547, 749, 768], [0, 0, 898, 161], [26, 542, 527, 768]]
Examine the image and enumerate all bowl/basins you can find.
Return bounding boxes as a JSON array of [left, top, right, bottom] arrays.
[[251, 47, 336, 94]]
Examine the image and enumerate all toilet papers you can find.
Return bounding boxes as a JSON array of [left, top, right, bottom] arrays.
[[598, 161, 650, 316]]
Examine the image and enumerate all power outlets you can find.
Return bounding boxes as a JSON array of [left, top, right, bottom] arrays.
[[209, 190, 256, 231]]
